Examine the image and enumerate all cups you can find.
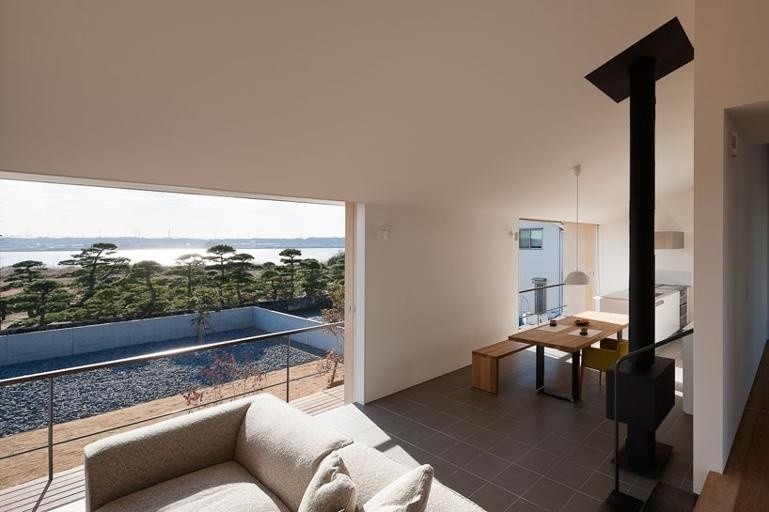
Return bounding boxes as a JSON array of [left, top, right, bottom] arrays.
[[581, 327, 587, 333], [550, 319, 556, 324]]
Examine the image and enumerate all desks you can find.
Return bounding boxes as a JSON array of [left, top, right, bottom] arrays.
[[508, 315, 628, 403]]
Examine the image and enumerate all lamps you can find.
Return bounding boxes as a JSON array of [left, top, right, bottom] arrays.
[[563, 164, 590, 286]]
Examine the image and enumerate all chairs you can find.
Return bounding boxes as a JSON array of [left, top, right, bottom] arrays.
[[581, 337, 628, 399]]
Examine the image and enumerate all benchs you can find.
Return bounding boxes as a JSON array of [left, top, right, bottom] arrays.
[[471, 338, 534, 395]]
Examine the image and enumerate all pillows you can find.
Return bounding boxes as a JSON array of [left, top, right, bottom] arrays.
[[297, 452, 436, 512]]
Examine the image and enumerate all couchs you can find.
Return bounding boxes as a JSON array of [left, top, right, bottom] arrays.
[[82, 389, 488, 512]]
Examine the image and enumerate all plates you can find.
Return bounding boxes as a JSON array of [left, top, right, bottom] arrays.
[[580, 332, 588, 335], [549, 323, 557, 326], [574, 319, 591, 325]]
